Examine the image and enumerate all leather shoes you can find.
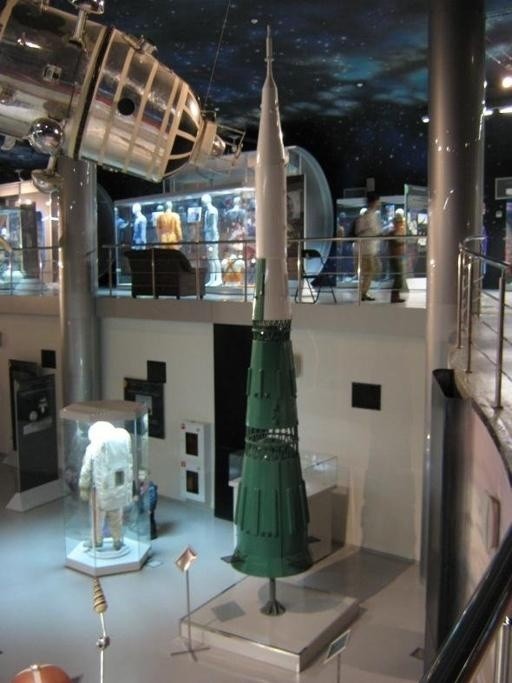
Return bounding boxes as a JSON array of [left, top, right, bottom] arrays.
[[362, 295, 375, 301], [391, 299, 406, 303]]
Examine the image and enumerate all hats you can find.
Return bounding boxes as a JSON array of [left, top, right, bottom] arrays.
[[396, 209, 404, 220]]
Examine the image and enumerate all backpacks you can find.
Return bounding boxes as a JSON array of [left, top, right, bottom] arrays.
[[346, 216, 368, 244]]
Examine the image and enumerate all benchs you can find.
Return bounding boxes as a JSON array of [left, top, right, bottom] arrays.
[[125, 248, 208, 299]]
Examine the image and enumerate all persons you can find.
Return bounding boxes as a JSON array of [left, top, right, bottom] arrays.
[[114, 194, 255, 287], [333, 192, 408, 303], [80, 421, 124, 550], [138, 468, 158, 539]]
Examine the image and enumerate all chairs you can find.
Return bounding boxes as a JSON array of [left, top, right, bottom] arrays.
[[294, 248, 338, 303]]
